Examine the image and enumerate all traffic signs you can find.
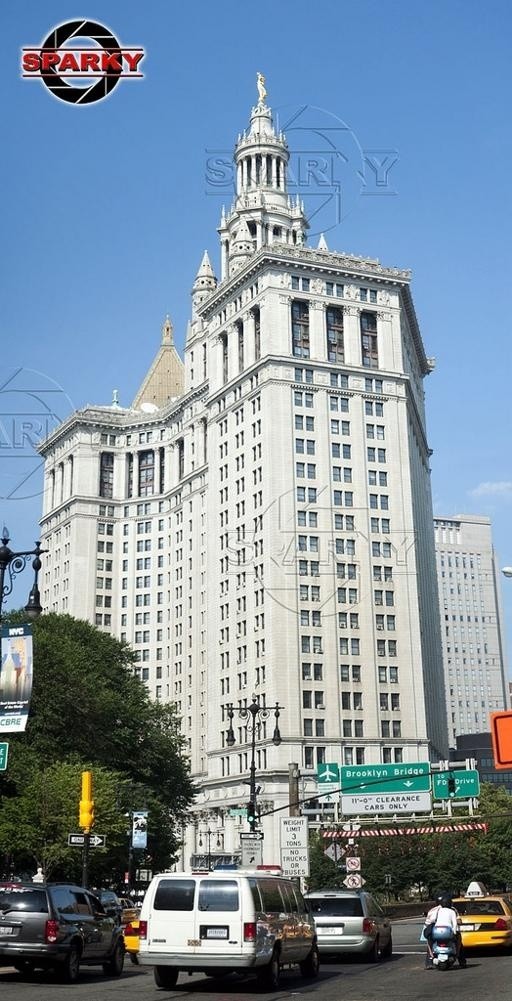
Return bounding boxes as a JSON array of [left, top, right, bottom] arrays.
[[317, 763, 339, 804], [341, 762, 433, 816], [433, 769, 480, 801], [68, 834, 106, 848], [240, 832, 264, 840], [1, 743, 9, 771]]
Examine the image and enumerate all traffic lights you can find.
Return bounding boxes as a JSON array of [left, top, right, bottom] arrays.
[[246, 804, 255, 823], [448, 778, 455, 797]]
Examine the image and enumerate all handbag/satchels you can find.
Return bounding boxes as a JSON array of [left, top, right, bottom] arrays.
[[424, 923, 435, 938]]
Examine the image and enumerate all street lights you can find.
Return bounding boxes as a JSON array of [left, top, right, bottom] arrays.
[[224, 693, 285, 831], [1, 528, 50, 671], [199, 829, 225, 869]]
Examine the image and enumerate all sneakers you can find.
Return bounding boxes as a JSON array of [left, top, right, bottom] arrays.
[[425, 965, 433, 971]]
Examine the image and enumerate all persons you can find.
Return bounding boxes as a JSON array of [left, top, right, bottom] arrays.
[[424, 896, 467, 969], [256, 71, 268, 106], [135, 896, 143, 908], [426, 897, 463, 959]]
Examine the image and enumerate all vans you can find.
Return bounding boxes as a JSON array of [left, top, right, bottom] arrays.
[[137, 865, 320, 992]]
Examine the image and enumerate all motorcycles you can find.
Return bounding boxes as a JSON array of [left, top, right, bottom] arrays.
[[431, 926, 457, 970]]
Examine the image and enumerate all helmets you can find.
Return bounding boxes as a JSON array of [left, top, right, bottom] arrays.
[[436, 895, 453, 908]]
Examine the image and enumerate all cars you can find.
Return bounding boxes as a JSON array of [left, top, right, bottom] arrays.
[[304, 892, 393, 964], [451, 882, 512, 957], [0, 883, 139, 982]]
